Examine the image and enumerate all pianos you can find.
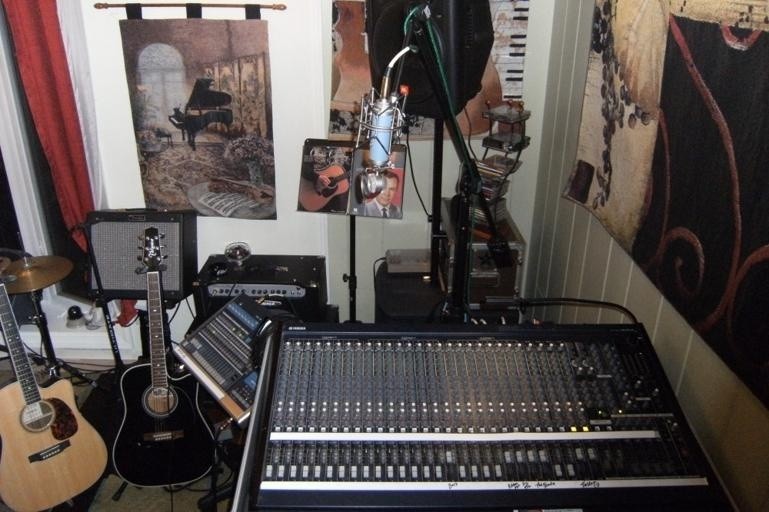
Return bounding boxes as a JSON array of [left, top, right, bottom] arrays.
[[169, 79, 233, 151]]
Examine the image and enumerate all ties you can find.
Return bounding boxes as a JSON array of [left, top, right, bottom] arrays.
[[381, 207, 388, 218]]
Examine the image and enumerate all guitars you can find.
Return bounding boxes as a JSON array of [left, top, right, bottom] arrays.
[[112, 225, 219, 488], [299, 163, 350, 211], [0, 269, 109, 512]]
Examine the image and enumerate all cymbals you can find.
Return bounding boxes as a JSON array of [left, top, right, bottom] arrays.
[[0, 255, 74, 293]]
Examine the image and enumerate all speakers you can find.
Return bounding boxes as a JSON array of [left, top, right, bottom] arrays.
[[192, 254, 340, 325], [374, 261, 443, 321]]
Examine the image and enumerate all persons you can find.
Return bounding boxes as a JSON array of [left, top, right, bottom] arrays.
[[364, 170, 402, 217], [302, 144, 353, 212]]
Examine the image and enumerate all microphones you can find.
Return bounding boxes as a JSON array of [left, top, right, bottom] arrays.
[[357, 99, 396, 198]]
